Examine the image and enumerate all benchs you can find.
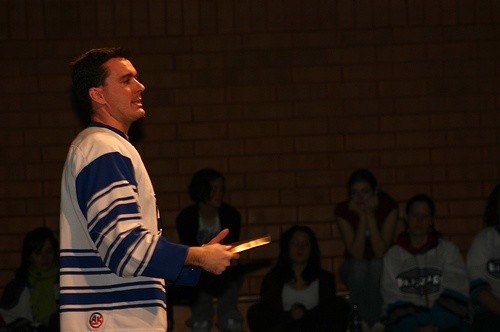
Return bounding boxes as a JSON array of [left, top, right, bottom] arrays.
[[170, 291, 350, 332]]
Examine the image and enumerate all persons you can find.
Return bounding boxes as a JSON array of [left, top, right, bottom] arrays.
[[59, 47, 240, 332], [0, 169, 500, 332]]
